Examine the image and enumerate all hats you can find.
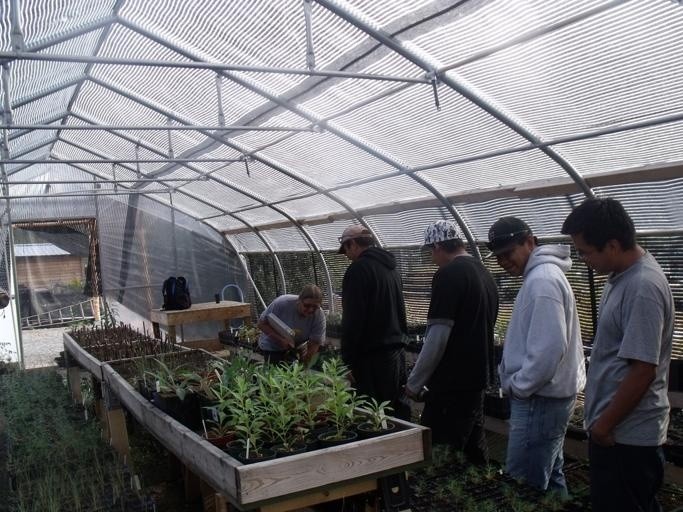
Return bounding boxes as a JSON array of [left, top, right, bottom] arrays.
[[419, 218, 463, 251], [338, 225, 373, 253], [485, 216, 532, 251]]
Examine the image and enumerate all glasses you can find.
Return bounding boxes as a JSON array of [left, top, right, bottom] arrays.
[[338, 235, 354, 243], [300, 300, 319, 309]]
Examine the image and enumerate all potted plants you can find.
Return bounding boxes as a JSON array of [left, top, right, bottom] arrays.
[[59, 318, 435, 512]]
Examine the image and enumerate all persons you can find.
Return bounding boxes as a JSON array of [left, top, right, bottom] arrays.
[[560, 198, 677, 510], [404, 216, 500, 466], [483, 216, 589, 499], [254, 282, 327, 372], [334, 224, 410, 417]]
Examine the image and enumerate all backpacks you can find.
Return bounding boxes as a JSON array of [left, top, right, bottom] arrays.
[[162, 276, 191, 310]]
